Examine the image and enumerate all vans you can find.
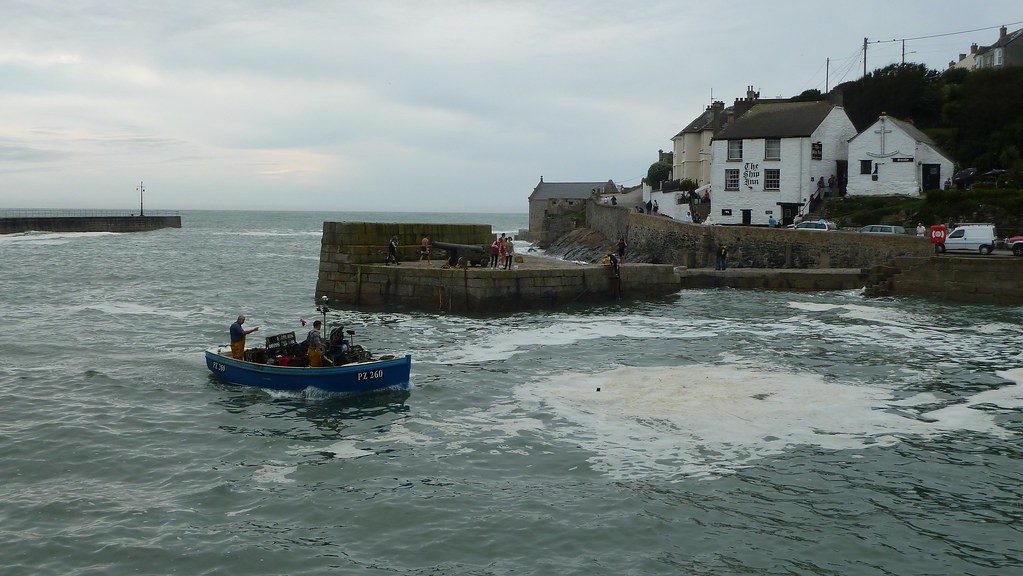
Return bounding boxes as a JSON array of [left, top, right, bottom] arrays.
[[936, 222, 998, 255]]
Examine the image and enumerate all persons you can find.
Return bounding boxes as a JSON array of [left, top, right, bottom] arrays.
[[611, 195, 617, 205], [686, 212, 691, 222], [944, 178, 951, 190], [635, 206, 644, 213], [817, 177, 825, 199], [949, 216, 964, 232], [386, 236, 400, 266], [653, 200, 658, 213], [646, 200, 652, 215], [491, 233, 515, 270], [307, 321, 322, 367], [828, 175, 834, 197], [420, 234, 432, 264], [916, 223, 926, 236], [616, 239, 627, 264], [715, 243, 727, 270], [691, 189, 709, 203], [604, 197, 608, 204], [230, 315, 259, 360], [769, 216, 781, 228]]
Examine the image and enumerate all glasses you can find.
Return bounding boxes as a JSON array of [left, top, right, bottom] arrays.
[[335, 332, 344, 337]]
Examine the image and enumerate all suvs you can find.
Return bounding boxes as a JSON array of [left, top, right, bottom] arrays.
[[855, 225, 908, 236], [788, 219, 840, 232]]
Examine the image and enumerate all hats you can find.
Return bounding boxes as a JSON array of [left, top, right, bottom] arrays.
[[918, 223, 921, 226], [330, 325, 344, 335], [604, 255, 608, 258]]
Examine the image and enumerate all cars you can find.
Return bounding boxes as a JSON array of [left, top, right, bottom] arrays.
[[1003, 236, 1023, 256]]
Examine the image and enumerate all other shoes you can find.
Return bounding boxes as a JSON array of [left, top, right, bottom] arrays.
[[386, 263, 390, 266], [495, 267, 498, 269], [490, 267, 493, 270], [503, 268, 506, 270], [396, 263, 401, 265]]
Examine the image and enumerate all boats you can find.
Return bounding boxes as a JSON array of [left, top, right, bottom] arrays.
[[205, 296, 412, 393]]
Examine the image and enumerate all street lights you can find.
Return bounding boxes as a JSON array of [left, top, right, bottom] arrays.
[[136, 181, 145, 217]]
